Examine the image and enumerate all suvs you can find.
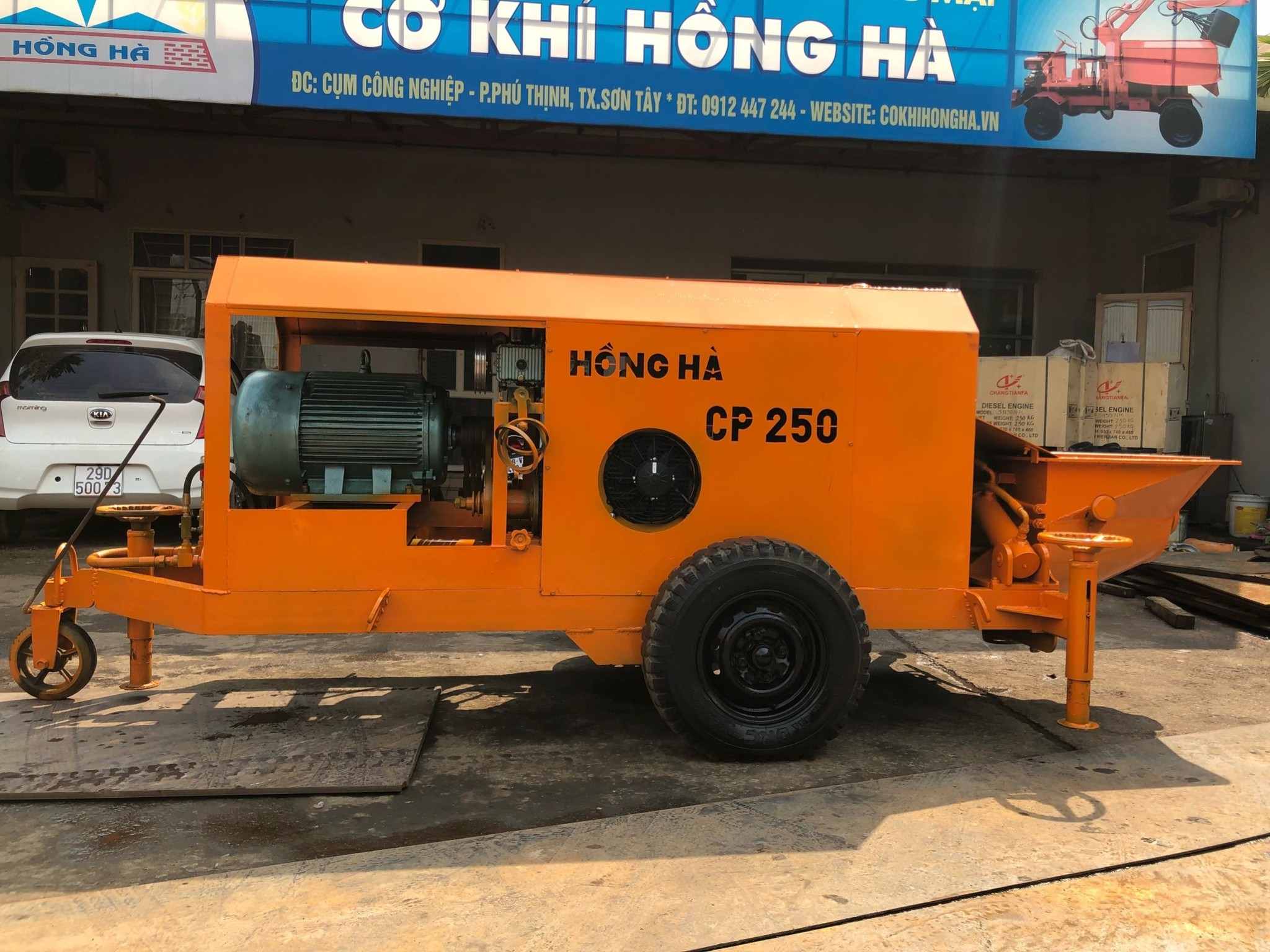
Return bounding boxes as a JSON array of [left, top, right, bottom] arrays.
[[0, 330, 248, 547]]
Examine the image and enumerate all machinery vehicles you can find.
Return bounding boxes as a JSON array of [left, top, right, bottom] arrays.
[[1010, 0, 1251, 148]]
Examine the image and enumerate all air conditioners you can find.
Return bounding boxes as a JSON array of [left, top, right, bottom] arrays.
[[1166, 177, 1254, 220], [14, 140, 105, 205]]
